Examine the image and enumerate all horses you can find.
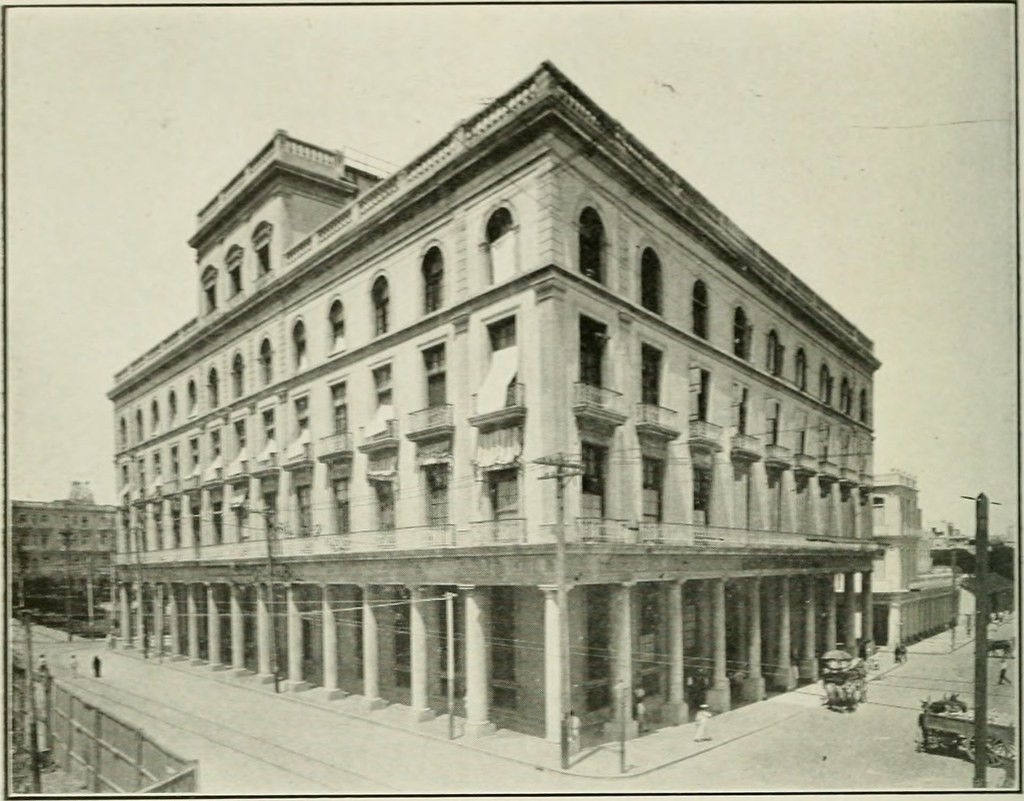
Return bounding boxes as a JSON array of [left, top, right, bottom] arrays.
[[987, 638, 1015, 658], [918, 691, 968, 751]]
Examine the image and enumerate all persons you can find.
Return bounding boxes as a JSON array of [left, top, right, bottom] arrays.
[[38, 655, 46, 674], [694, 705, 710, 741], [998, 660, 1011, 685], [895, 643, 907, 663], [635, 700, 647, 731], [70, 655, 77, 673], [93, 656, 101, 678], [570, 711, 582, 752]]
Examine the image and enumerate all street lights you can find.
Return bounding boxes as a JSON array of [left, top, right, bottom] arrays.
[[231, 505, 280, 694]]
